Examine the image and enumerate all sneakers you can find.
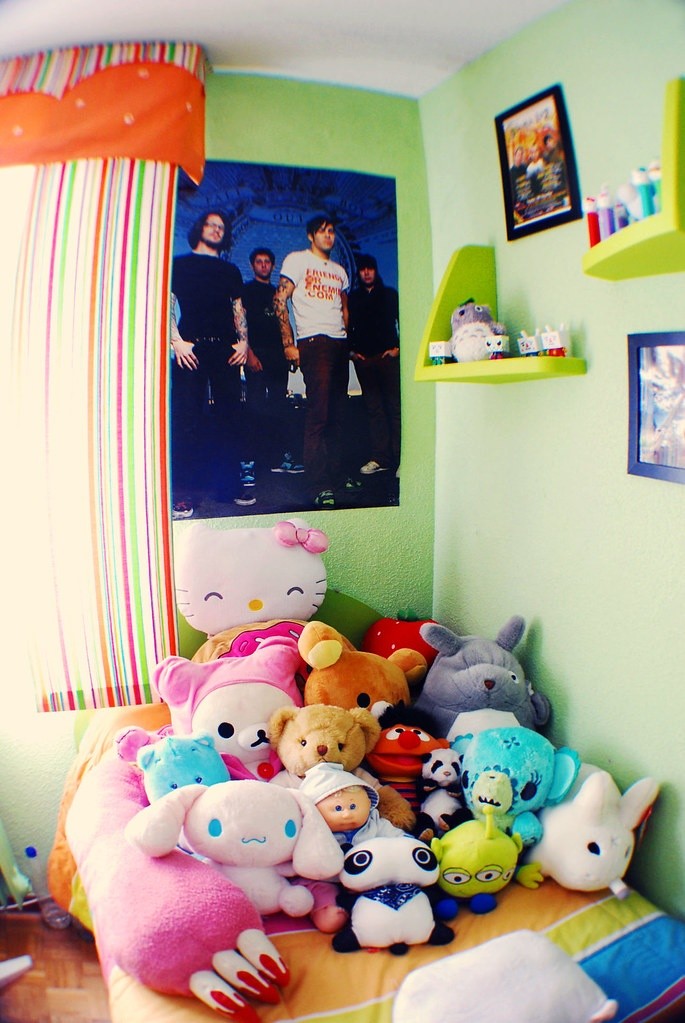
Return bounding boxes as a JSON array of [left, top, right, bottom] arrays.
[[230, 489, 256, 506], [171, 495, 194, 518], [360, 460, 391, 474], [312, 489, 335, 509], [270, 450, 305, 474], [335, 477, 372, 492], [239, 459, 256, 487]]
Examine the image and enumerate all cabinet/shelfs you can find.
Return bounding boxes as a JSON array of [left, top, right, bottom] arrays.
[[411, 75, 685, 387]]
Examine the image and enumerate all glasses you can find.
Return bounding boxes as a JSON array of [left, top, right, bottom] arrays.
[[203, 221, 225, 230]]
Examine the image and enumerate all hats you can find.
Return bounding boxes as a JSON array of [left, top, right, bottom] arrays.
[[357, 254, 376, 270]]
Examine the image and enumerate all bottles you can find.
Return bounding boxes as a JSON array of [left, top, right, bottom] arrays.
[[584, 160, 661, 250], [25, 844, 69, 929]]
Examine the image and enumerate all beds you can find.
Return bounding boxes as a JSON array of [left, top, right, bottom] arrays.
[[46, 702, 685, 1022]]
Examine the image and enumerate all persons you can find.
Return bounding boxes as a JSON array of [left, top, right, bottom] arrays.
[[242, 247, 306, 487], [346, 253, 400, 481], [274, 215, 366, 506], [509, 133, 570, 223], [171, 210, 257, 519]]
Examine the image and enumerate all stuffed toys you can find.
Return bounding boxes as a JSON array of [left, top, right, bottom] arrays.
[[449, 301, 506, 365], [60, 520, 663, 1022]]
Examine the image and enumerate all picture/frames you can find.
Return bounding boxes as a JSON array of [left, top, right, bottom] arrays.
[[493, 83, 582, 242], [624, 329, 684, 486]]
